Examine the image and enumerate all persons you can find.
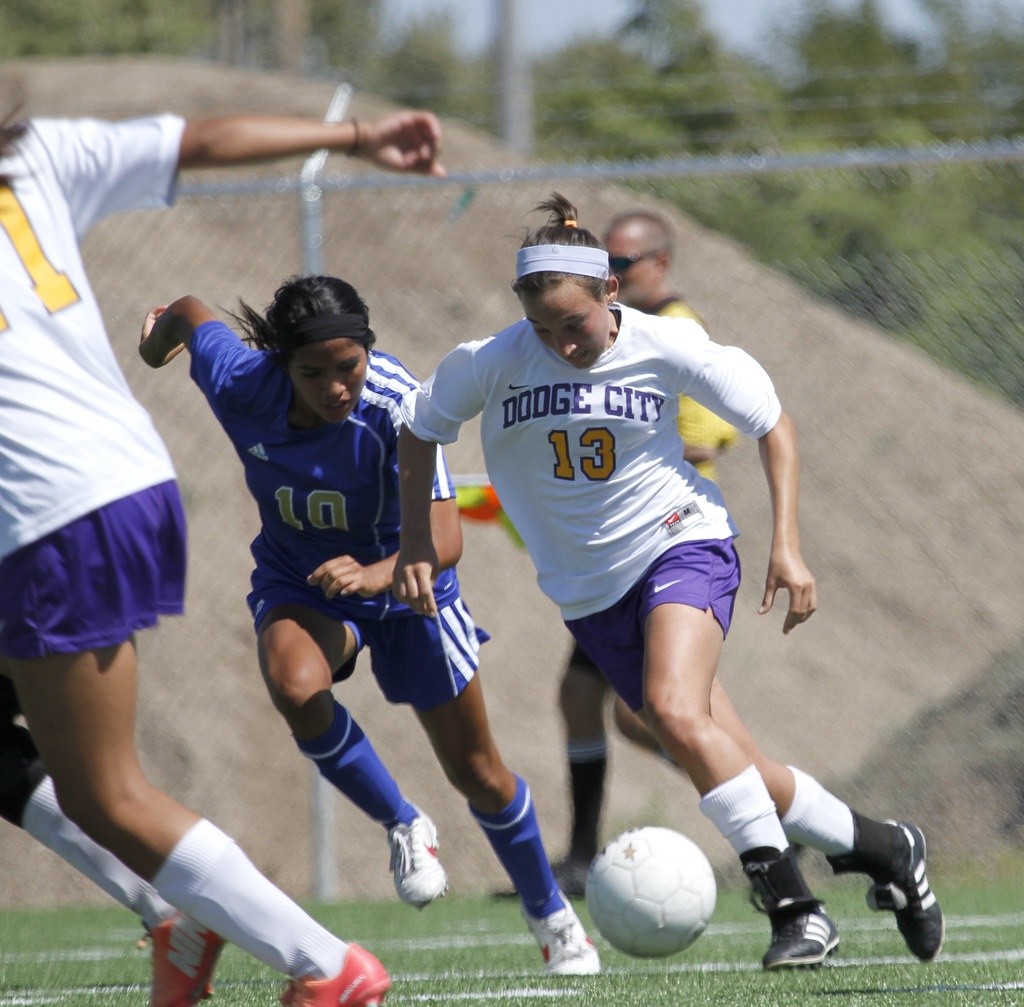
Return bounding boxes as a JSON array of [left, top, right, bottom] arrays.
[[0, 60, 456, 1007], [138, 275, 606, 978], [392, 190, 953, 974], [489, 208, 810, 903]]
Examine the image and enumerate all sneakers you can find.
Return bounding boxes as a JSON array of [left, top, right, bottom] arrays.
[[138, 912, 227, 1007], [521, 892, 601, 976], [865, 819, 944, 961], [280, 942, 391, 1007], [386, 796, 449, 909], [549, 857, 589, 901], [761, 896, 841, 968]]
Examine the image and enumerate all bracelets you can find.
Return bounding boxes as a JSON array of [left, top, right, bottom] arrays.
[[348, 116, 366, 159]]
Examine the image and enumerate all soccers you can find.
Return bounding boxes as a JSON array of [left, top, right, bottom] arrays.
[[584, 823, 719, 960]]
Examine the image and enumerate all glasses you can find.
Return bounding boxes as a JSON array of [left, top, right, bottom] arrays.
[[608, 252, 654, 271]]
